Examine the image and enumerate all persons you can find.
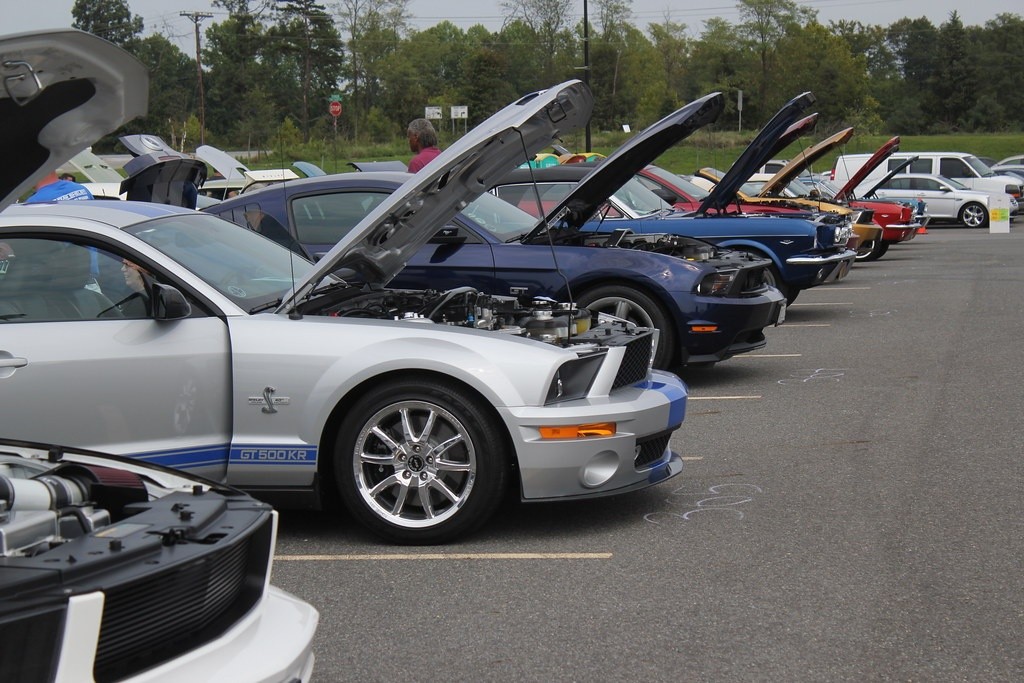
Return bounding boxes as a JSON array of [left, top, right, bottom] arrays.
[[406, 118, 441, 173], [206, 167, 238, 200], [23, 170, 99, 293], [121, 258, 152, 296]]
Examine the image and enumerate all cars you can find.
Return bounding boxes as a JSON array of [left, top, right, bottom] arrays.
[[0, 28, 320, 681], [49, 133, 329, 228], [287, 90, 857, 314], [126, 92, 787, 378], [356, 92, 1024, 301], [0, 27, 691, 546]]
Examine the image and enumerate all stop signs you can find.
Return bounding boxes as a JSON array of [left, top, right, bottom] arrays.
[[328, 101, 342, 117]]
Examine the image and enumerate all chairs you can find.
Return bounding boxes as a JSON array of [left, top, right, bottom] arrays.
[[0, 253, 70, 321], [46, 246, 127, 321]]
